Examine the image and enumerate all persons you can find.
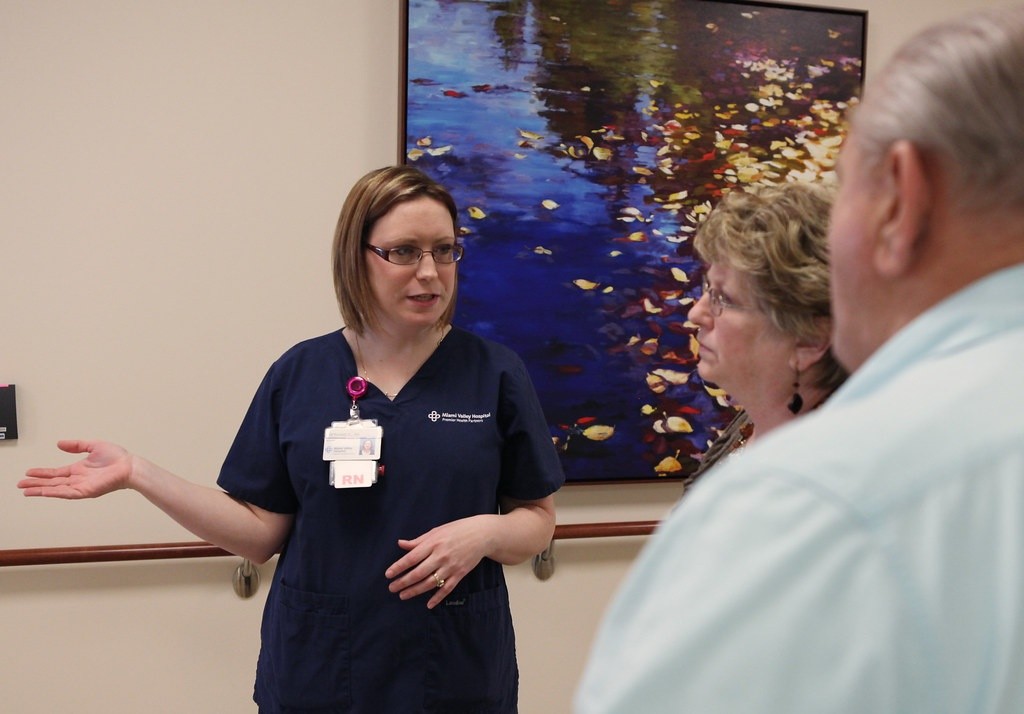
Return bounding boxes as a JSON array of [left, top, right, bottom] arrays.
[[572, 15, 1023, 714], [17, 165, 565, 714], [676, 176, 851, 487]]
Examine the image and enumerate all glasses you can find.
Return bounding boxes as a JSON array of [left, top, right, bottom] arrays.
[[366, 243, 464, 265], [702, 275, 761, 316]]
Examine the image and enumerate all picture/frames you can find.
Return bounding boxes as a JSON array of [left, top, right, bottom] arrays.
[[398, 0, 867, 487]]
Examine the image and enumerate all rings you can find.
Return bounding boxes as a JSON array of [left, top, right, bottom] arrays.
[[434, 572, 446, 587]]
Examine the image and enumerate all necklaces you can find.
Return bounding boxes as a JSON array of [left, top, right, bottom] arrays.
[[354, 323, 444, 398]]
